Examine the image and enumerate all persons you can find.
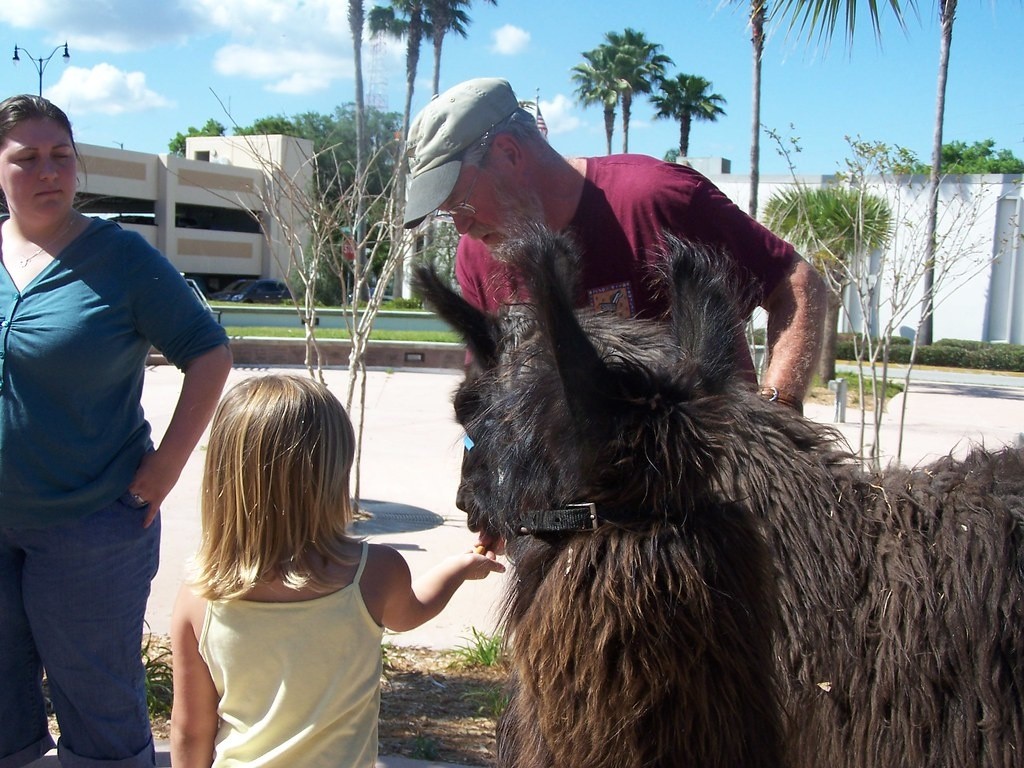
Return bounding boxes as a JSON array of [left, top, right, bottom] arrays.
[[404, 79, 826, 418], [0, 94, 234, 768], [170, 374, 506, 768]]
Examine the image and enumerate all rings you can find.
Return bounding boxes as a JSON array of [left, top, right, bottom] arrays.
[[136, 494, 145, 504]]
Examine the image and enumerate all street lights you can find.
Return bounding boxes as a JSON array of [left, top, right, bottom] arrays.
[[11, 42, 71, 98]]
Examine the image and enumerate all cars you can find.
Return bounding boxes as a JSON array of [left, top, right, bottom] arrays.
[[207, 279, 304, 304]]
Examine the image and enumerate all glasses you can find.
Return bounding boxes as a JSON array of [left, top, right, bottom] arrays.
[[435, 141, 494, 222]]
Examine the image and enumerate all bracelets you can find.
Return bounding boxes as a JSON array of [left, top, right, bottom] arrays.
[[755, 385, 802, 413]]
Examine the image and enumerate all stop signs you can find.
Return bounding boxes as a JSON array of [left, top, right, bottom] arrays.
[[342, 236, 358, 260]]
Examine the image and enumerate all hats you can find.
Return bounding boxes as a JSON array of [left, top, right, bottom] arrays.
[[403, 78, 519, 229]]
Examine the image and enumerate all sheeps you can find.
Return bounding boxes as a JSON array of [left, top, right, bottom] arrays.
[[407, 219, 1024, 768]]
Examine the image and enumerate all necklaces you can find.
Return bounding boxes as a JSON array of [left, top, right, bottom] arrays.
[[18, 212, 77, 267]]
